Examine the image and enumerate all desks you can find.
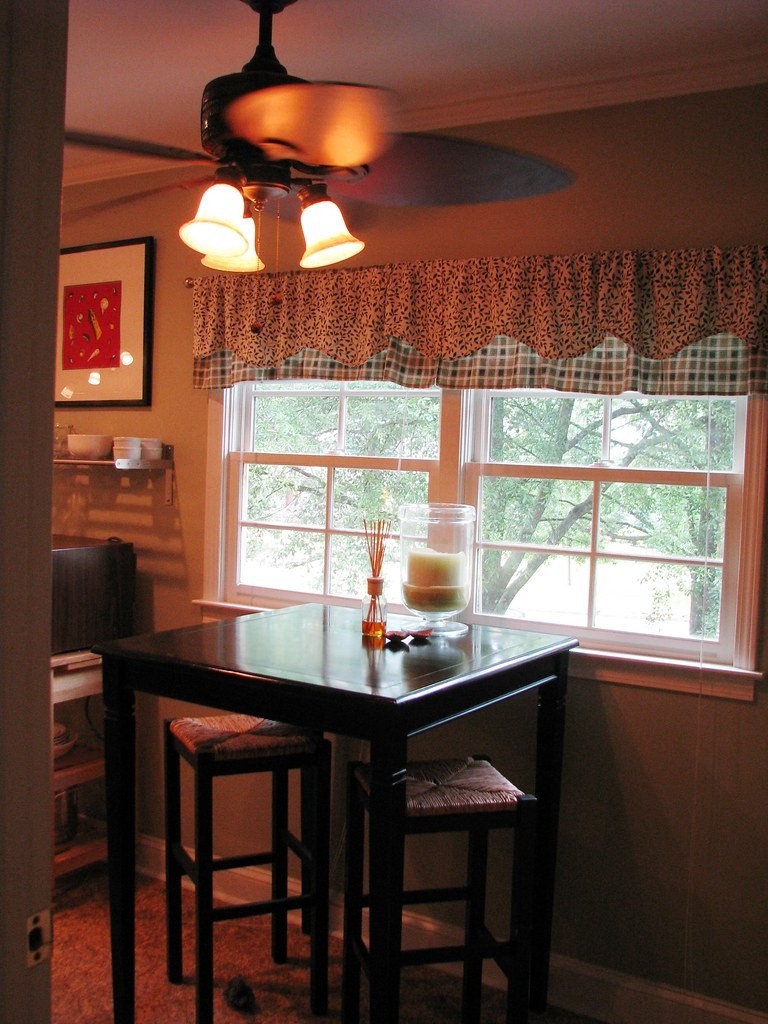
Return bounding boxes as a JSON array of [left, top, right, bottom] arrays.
[[91, 601, 582, 1024]]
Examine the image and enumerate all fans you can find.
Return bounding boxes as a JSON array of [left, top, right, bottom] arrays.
[[59, 80, 576, 229]]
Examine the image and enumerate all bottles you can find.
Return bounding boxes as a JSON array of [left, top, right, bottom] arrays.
[[361, 578, 387, 639]]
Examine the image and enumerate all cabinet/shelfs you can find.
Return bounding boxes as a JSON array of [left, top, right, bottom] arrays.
[[51, 645, 143, 883]]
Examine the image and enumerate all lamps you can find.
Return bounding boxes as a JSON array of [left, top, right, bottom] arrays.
[[179, 1, 365, 335]]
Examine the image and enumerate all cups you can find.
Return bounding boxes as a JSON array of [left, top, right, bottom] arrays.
[[397, 502, 477, 637]]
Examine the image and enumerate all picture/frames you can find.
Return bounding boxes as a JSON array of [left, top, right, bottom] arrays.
[[53, 235, 156, 407]]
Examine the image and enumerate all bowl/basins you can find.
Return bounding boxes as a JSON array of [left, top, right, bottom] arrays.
[[112, 447, 142, 461], [68, 434, 112, 460], [113, 436, 141, 447], [143, 448, 163, 460], [140, 438, 161, 448]]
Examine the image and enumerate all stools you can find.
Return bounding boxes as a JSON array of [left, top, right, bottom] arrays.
[[162, 715, 334, 1024], [342, 755, 538, 1024]]
